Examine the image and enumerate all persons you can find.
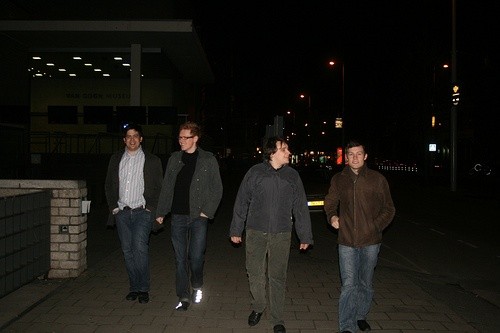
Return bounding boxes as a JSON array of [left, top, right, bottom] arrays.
[[154, 121, 224, 311], [324, 142, 396, 333], [105, 125, 164, 302], [228, 136, 314, 333]]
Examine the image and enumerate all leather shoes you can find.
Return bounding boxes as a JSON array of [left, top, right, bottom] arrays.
[[357, 320, 372, 331], [125, 291, 138, 301], [341, 330, 352, 333], [138, 290, 149, 304], [274, 325, 286, 333], [248, 310, 263, 326]]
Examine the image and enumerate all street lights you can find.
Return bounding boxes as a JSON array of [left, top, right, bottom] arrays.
[[329, 60, 346, 128], [287, 108, 295, 130], [300, 94, 311, 113]]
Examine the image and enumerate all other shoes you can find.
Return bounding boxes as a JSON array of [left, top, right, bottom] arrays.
[[175, 299, 188, 312], [190, 288, 202, 304]]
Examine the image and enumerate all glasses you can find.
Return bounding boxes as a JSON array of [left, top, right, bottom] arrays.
[[345, 151, 366, 158], [177, 135, 195, 141], [126, 134, 139, 140]]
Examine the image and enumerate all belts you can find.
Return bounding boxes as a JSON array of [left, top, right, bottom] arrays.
[[123, 206, 145, 212]]
